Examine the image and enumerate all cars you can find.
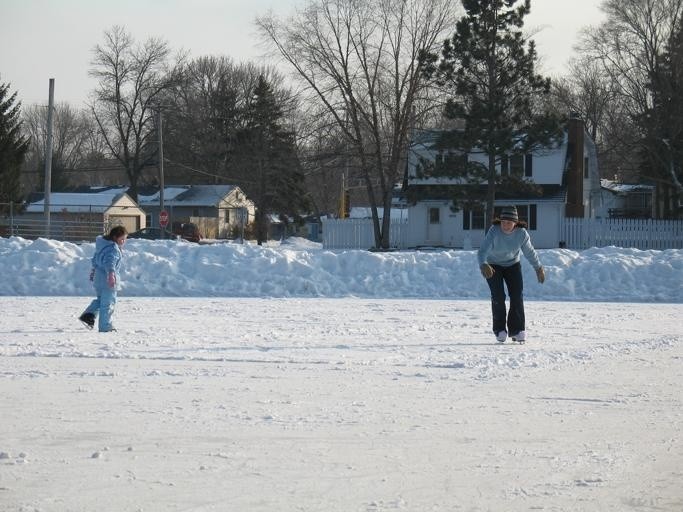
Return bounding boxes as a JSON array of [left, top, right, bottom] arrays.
[[126, 221, 203, 242]]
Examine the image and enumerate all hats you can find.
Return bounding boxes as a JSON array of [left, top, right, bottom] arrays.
[[498, 204, 519, 223]]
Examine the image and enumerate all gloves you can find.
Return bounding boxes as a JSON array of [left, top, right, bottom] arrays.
[[89, 269, 96, 282], [535, 265, 546, 284], [108, 271, 116, 288], [480, 263, 496, 279]]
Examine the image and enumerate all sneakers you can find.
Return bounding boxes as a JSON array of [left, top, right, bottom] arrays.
[[510, 330, 526, 341], [496, 330, 507, 342], [79, 312, 95, 326], [99, 329, 117, 332]]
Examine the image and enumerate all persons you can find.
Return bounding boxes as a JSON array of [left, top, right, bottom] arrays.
[[477, 206, 544, 341], [79, 226, 128, 333]]
